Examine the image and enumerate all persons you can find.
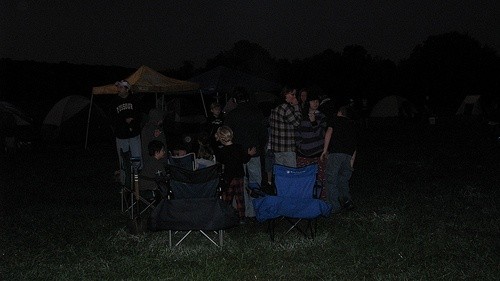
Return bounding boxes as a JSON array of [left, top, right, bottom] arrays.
[[297, 87, 310, 118], [214, 125, 256, 224], [111, 79, 144, 177], [202, 103, 226, 158], [268, 84, 300, 167], [137, 138, 170, 212], [317, 95, 337, 160], [296, 96, 328, 157]]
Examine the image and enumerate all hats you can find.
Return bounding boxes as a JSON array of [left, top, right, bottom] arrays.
[[114, 79, 131, 89]]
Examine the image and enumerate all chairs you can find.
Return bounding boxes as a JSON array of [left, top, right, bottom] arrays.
[[132, 153, 331, 250]]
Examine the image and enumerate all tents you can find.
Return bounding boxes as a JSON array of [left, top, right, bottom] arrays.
[[84, 64, 208, 151]]
